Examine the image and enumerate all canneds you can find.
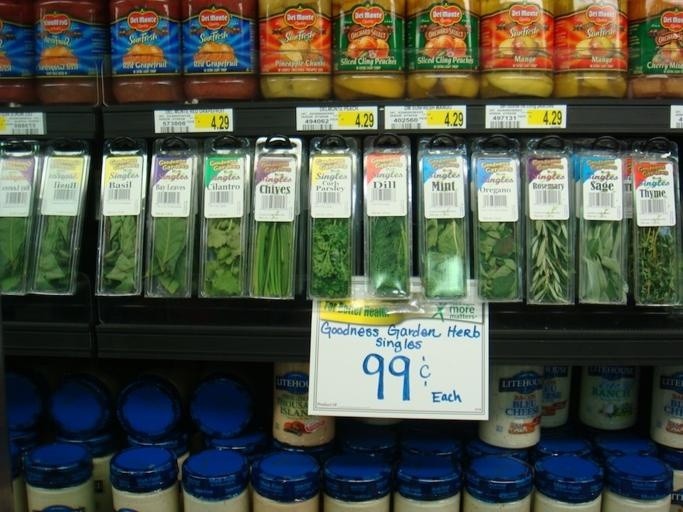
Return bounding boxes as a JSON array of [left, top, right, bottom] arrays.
[[481, 0, 555, 99], [111, 1, 184, 104], [36, 1, 110, 104], [184, 1, 259, 102], [628, 1, 683, 100], [334, 0, 407, 101], [556, 0, 629, 99], [1, 1, 36, 105], [258, 1, 334, 101], [406, 1, 481, 100]]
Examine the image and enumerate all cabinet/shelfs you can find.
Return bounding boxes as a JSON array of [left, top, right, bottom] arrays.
[[0, 98, 683, 366]]
